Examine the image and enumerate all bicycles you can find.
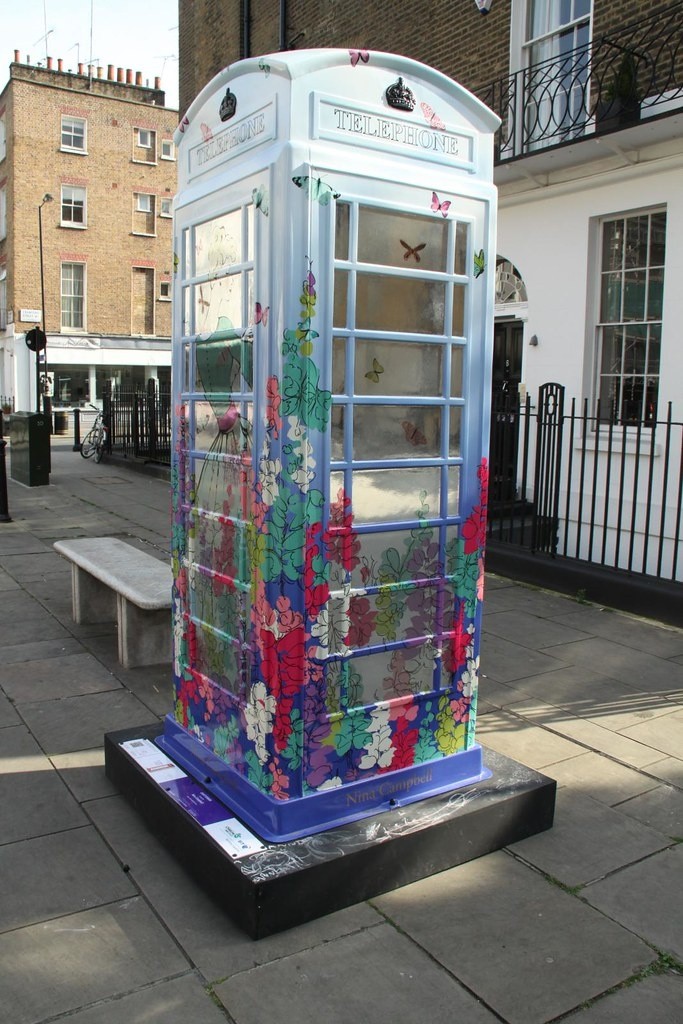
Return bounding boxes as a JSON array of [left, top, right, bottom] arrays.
[[79, 404, 109, 464]]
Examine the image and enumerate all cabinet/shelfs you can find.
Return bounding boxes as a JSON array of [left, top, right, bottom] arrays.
[[59, 376, 71, 401]]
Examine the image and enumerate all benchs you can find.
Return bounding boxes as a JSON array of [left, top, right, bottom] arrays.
[[54, 537, 174, 669]]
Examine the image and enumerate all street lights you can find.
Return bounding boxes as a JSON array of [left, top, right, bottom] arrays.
[[38, 193, 55, 438]]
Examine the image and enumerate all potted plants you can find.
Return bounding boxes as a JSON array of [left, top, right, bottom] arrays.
[[595, 46, 641, 131], [2, 403, 11, 414]]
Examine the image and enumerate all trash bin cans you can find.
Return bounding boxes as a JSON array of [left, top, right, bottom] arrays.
[[54, 411, 69, 435], [9, 411, 50, 488]]
